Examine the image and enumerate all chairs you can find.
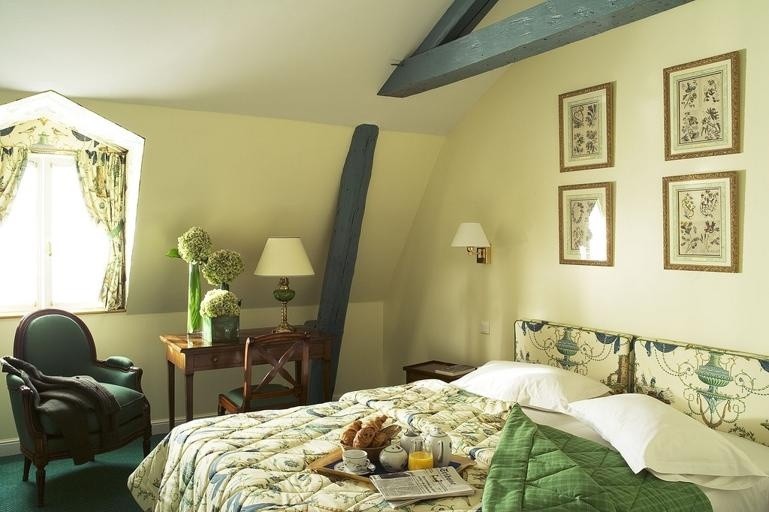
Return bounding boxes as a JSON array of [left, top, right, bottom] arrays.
[[218, 334, 313, 420], [1, 308, 154, 511]]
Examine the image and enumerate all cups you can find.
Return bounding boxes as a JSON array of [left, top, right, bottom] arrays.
[[409, 442, 433, 469], [341, 449, 371, 472], [400, 432, 423, 452]]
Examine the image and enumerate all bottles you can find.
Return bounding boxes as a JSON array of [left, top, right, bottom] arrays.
[[378, 440, 407, 471]]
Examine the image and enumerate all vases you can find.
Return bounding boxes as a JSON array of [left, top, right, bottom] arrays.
[[204, 315, 239, 344], [184, 260, 204, 338]]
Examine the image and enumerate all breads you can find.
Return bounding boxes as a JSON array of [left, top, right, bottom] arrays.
[[340, 414, 398, 448]]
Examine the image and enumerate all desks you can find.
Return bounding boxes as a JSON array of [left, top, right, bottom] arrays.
[[155, 323, 337, 437]]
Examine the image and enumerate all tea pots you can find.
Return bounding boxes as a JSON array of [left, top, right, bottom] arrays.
[[424, 424, 452, 468]]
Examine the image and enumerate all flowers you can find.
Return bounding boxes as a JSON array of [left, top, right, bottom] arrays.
[[165, 222, 213, 333], [201, 246, 245, 292], [199, 284, 242, 322]]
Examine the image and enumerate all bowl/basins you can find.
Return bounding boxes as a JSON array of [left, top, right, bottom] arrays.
[[339, 439, 392, 461]]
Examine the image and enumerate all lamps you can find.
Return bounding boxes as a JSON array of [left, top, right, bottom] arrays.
[[450, 220, 490, 267], [251, 235, 314, 334]]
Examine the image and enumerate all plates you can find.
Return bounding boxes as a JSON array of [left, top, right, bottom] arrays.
[[334, 462, 376, 475]]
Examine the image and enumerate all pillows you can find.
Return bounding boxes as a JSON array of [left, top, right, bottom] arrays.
[[561, 391, 768, 490], [449, 356, 619, 417]]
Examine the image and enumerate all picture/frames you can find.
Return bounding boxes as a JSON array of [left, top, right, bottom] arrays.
[[558, 180, 616, 269], [558, 83, 615, 178], [661, 167, 741, 273], [664, 47, 742, 163]]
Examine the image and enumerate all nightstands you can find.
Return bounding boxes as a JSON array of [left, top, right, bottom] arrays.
[[402, 359, 476, 386]]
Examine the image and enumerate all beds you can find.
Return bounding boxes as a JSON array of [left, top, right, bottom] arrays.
[[124, 318, 769, 512]]
